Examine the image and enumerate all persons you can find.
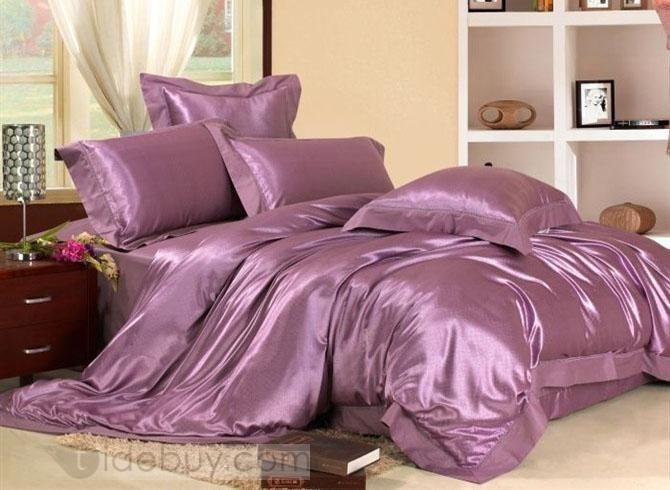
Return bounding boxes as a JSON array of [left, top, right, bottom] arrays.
[[584, 88, 607, 123]]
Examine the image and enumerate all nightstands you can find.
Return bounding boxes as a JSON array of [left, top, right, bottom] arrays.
[[0, 251, 98, 381]]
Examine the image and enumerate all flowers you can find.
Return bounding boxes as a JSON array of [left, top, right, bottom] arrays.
[[0, 221, 118, 294]]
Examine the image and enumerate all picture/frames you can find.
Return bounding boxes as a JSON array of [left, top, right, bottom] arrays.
[[581, 0, 612, 10], [575, 79, 615, 129], [620, 0, 647, 10], [468, 0, 506, 12]]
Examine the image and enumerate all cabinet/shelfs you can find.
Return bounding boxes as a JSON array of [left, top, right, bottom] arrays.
[[454, 0, 670, 250]]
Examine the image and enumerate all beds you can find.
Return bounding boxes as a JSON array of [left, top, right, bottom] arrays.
[[0, 221, 670, 485]]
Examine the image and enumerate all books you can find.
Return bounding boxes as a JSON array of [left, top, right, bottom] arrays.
[[529, 0, 537, 12], [258, 432, 382, 477], [613, 119, 670, 127], [610, 126, 665, 130], [545, 0, 553, 12], [536, 0, 545, 11], [260, 460, 367, 490]]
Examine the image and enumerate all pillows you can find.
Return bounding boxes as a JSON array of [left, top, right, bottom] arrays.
[[19, 217, 105, 245], [340, 163, 583, 257], [139, 69, 301, 142], [203, 120, 394, 220], [52, 114, 232, 253]]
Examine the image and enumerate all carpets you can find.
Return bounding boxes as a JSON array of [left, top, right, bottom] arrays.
[[53, 427, 415, 490]]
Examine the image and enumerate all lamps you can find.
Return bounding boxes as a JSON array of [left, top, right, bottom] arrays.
[[1, 122, 46, 261]]
